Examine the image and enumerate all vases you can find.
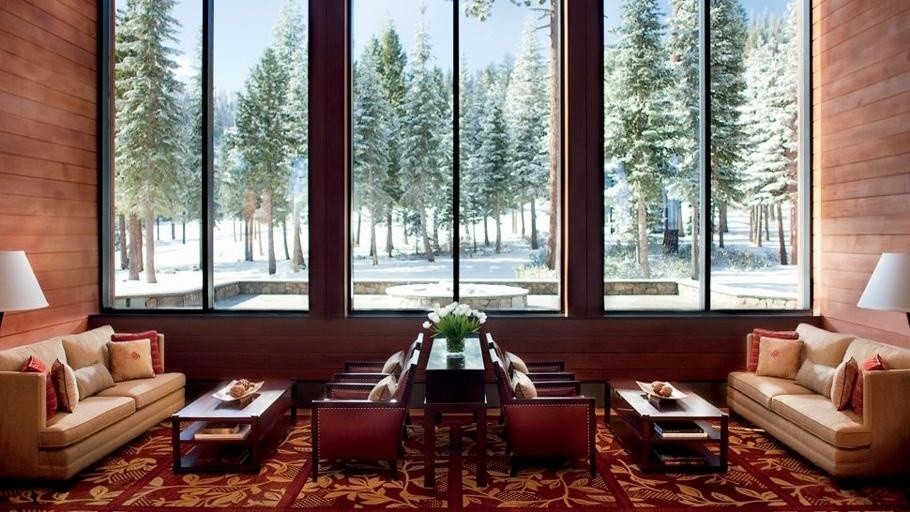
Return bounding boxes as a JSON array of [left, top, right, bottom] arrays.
[[446, 332, 466, 360]]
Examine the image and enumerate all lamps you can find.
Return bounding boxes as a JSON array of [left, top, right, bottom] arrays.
[[857, 248, 910, 329], [1, 249, 49, 328]]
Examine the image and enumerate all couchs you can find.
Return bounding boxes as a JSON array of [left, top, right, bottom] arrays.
[[1, 322, 188, 492], [725, 321, 910, 491]]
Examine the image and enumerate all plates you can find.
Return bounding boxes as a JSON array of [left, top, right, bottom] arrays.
[[635, 379, 687, 399], [213, 379, 265, 403]]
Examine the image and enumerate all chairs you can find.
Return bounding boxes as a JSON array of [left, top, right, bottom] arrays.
[[486, 332, 576, 381], [489, 348, 598, 480], [333, 331, 425, 383], [312, 348, 420, 483]]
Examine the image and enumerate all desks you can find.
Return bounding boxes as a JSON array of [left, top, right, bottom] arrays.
[[422, 332, 488, 488]]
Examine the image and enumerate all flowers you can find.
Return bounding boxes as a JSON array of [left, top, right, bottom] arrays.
[[421, 300, 489, 352]]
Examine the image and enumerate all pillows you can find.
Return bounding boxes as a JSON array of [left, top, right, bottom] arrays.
[[381, 348, 406, 374], [503, 350, 531, 378], [512, 368, 538, 399], [367, 373, 399, 401], [17, 328, 165, 415], [748, 326, 888, 417]]
